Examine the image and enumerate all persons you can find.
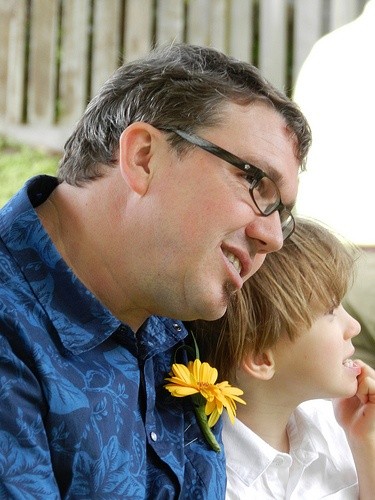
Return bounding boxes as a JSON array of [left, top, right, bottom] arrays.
[[193, 216, 375, 500], [1, 45, 309, 500]]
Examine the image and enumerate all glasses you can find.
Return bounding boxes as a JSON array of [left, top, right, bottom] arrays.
[[174, 127, 296, 243]]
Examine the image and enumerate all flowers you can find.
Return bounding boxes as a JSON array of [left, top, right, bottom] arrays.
[[162, 329, 246, 452]]
